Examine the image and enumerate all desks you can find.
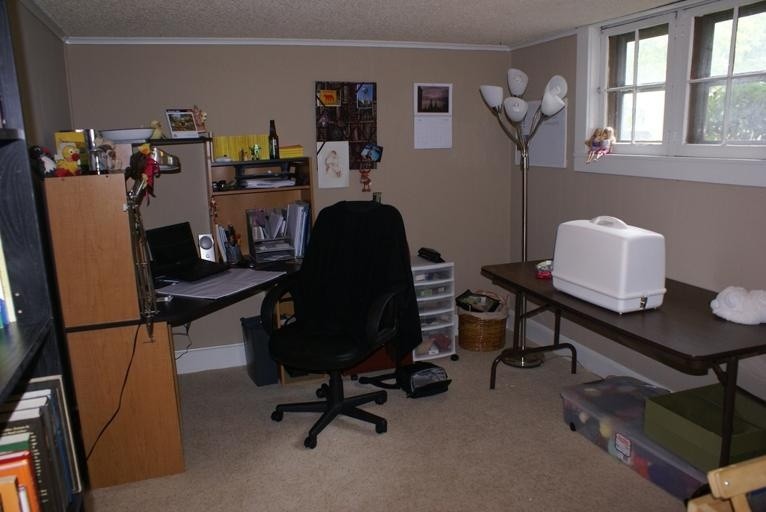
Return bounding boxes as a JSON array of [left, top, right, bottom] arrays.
[[481, 260, 766, 468], [44, 136, 330, 490]]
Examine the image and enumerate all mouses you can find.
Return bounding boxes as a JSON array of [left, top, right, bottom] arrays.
[[248, 256, 258, 270]]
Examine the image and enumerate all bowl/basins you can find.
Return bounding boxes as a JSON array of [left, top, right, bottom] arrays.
[[98, 129, 155, 144]]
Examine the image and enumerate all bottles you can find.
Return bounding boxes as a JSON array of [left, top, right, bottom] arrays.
[[268, 119, 278, 159]]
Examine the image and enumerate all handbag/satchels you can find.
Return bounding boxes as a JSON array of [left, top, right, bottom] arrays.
[[394, 361, 453, 399]]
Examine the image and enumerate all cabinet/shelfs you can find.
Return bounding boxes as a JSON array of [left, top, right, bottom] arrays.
[[411, 256, 459, 361], [0, 0, 87, 512]]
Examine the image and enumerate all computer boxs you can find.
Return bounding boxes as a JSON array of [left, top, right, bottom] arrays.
[[241, 314, 279, 386]]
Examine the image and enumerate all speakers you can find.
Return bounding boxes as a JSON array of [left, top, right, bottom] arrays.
[[198, 233, 215, 263]]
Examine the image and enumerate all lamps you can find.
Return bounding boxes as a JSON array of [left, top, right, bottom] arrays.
[[479, 67, 577, 388], [126, 145, 182, 338]]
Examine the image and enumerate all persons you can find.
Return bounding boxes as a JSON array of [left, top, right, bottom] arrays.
[[326, 150, 341, 177], [584, 126, 616, 164]]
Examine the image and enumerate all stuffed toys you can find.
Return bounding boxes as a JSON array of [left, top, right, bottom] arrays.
[[53, 145, 82, 178], [29, 143, 56, 181], [150, 120, 165, 140]]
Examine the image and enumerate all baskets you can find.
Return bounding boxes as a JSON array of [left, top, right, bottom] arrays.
[[459, 310, 508, 353]]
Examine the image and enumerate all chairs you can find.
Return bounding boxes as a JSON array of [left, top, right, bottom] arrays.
[[706, 455, 766, 512], [261, 201, 422, 448]]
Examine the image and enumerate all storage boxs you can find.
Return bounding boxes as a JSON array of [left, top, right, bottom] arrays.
[[552, 216, 667, 316], [560, 375, 766, 501]]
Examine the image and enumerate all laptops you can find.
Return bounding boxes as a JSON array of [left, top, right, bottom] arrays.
[[145, 221, 231, 281]]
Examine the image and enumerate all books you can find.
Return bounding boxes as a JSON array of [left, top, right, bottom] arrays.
[[215, 224, 229, 262], [0, 235, 84, 511], [251, 198, 312, 264]]
